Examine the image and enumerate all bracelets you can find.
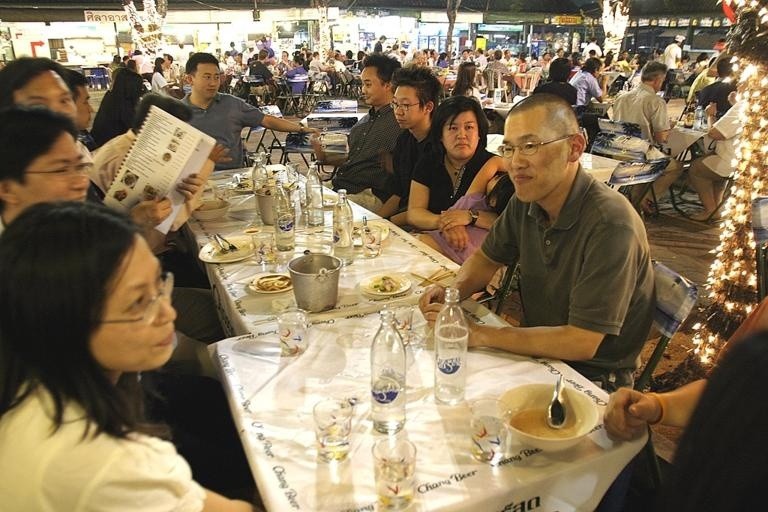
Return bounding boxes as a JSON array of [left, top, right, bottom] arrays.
[[645, 391, 665, 428], [300, 122, 306, 133]]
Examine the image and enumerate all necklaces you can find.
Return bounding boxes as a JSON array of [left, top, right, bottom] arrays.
[[446, 158, 464, 177]]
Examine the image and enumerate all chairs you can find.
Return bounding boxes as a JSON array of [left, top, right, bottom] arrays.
[[311, 99, 359, 115], [334, 70, 362, 105], [240, 74, 276, 106], [249, 104, 311, 168], [590, 131, 651, 206], [597, 116, 666, 228], [634, 257, 701, 393], [284, 80, 308, 116]]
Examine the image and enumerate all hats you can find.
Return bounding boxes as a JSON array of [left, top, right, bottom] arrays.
[[675, 35, 686, 42]]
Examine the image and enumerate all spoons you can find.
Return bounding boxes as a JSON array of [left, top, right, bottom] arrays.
[[545, 374, 568, 429], [214, 234, 238, 254]]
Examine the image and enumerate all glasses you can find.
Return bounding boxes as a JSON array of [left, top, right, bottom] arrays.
[[18, 161, 94, 179], [390, 100, 420, 111], [100, 271, 175, 325], [502, 133, 577, 158]]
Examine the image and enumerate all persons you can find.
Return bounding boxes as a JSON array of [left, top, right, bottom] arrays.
[[603, 293, 768, 512], [215, 37, 362, 100], [533, 58, 577, 105], [152, 57, 179, 98], [484, 50, 514, 88], [328, 50, 407, 212], [659, 35, 687, 104], [0, 57, 227, 340], [170, 53, 320, 171], [407, 96, 507, 250], [0, 200, 264, 512], [375, 66, 443, 232], [574, 36, 664, 74], [89, 92, 234, 232], [450, 62, 486, 109], [614, 62, 682, 219], [690, 91, 747, 222], [679, 39, 728, 77], [707, 49, 734, 77], [505, 48, 565, 72], [566, 58, 609, 132], [417, 94, 655, 394], [697, 57, 740, 116], [373, 35, 486, 82], [62, 66, 95, 131], [414, 156, 515, 269], [686, 57, 717, 104], [0, 105, 88, 231], [106, 48, 174, 94]]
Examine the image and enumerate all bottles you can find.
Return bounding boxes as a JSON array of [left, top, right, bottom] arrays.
[[433, 288, 466, 403], [306, 162, 323, 226], [371, 312, 406, 435], [253, 160, 268, 216], [333, 190, 353, 251], [274, 183, 296, 253]]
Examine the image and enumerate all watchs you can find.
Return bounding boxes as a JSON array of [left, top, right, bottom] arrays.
[[466, 207, 481, 227]]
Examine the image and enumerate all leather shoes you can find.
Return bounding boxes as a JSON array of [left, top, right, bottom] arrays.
[[691, 211, 720, 221], [640, 200, 657, 214]]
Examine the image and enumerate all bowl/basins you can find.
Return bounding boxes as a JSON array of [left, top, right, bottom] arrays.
[[497, 384, 602, 451], [191, 200, 232, 222]]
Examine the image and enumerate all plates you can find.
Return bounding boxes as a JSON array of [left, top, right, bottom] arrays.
[[350, 218, 389, 247], [248, 273, 293, 294], [201, 235, 257, 264], [301, 189, 338, 208], [360, 273, 412, 296], [233, 179, 261, 193]]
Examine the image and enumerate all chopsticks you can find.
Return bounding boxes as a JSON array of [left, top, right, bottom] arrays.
[[411, 266, 456, 288]]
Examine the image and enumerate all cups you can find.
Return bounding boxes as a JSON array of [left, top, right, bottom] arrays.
[[469, 398, 511, 465], [285, 161, 299, 185], [388, 304, 415, 347], [360, 226, 382, 258], [277, 309, 308, 355], [254, 232, 274, 263], [313, 398, 353, 464], [371, 437, 416, 509]]
